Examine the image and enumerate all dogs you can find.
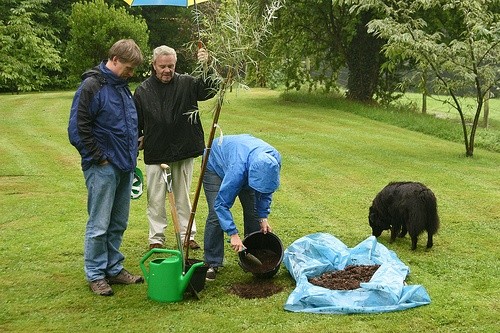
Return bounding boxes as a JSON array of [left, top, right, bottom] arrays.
[[368, 181, 440, 251]]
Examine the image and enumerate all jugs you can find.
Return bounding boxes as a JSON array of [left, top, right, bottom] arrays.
[[139, 248, 205, 302]]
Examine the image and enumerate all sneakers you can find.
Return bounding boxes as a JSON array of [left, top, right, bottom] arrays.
[[106, 268, 142, 284], [89, 278, 113, 295]]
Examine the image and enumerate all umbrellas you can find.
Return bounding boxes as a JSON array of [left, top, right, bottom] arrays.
[[125, 0, 209, 51]]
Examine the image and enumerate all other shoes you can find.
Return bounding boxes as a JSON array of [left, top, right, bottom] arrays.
[[206, 268, 216, 281], [150, 244, 162, 249], [184, 240, 200, 249]]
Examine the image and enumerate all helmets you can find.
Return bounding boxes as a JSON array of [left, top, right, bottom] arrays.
[[131, 167, 143, 199]]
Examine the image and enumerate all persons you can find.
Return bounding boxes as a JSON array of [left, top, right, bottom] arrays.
[[68, 39, 144, 296], [202, 133, 282, 280], [133, 46, 222, 252]]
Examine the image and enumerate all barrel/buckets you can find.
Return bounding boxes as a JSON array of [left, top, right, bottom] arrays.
[[186, 258, 211, 299], [238, 229, 284, 279]]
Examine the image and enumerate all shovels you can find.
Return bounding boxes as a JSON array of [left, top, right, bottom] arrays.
[[227, 240, 263, 266], [160, 164, 211, 300]]
